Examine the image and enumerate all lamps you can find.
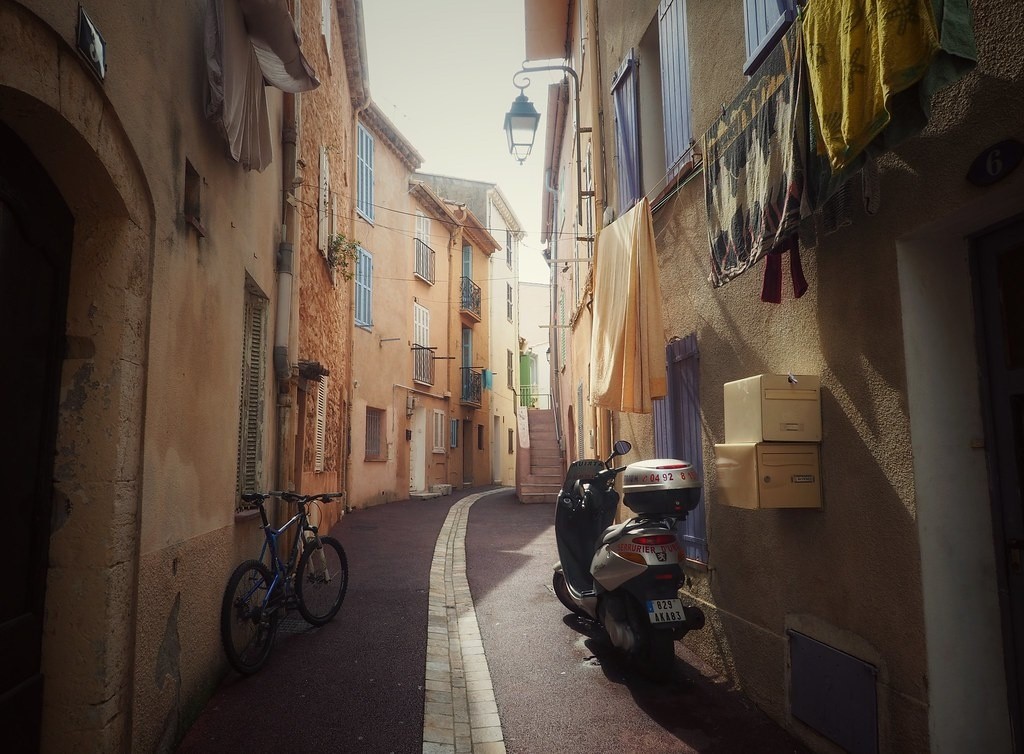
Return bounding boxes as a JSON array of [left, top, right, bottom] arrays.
[[503, 58, 596, 225]]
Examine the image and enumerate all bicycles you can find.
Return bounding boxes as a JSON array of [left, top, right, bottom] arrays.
[[220, 489, 349, 677]]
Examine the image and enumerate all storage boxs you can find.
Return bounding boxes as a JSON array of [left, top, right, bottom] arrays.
[[714, 444, 824, 513], [723, 375, 824, 444]]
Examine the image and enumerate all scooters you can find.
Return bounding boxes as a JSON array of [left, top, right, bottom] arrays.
[[551, 440, 706, 687]]
